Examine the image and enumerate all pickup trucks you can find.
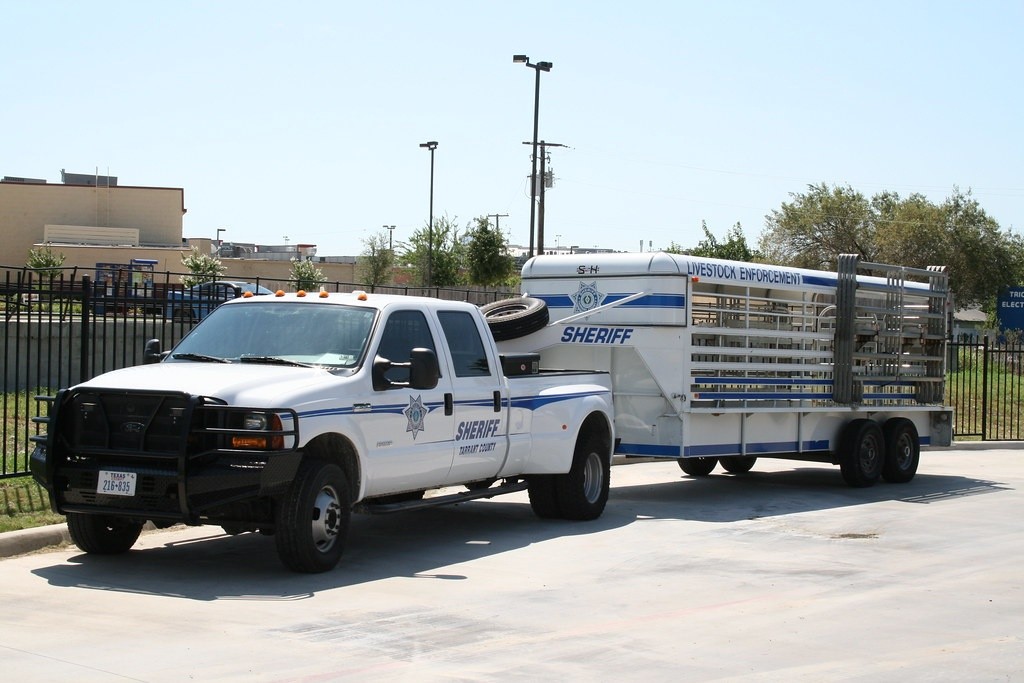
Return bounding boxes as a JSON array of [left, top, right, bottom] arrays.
[[31, 289, 621, 572]]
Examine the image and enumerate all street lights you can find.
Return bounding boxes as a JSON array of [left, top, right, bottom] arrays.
[[511, 54, 554, 260], [215, 228, 225, 254], [419, 140, 439, 298], [383, 225, 396, 251]]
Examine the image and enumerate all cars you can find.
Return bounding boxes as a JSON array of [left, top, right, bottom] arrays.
[[165, 280, 275, 323]]
